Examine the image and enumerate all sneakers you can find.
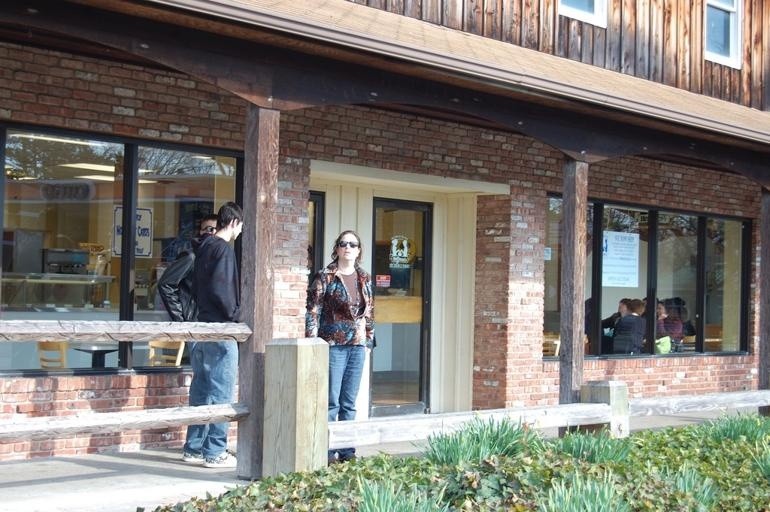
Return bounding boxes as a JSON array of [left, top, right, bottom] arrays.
[[183, 452, 204, 462], [204, 452, 237, 468]]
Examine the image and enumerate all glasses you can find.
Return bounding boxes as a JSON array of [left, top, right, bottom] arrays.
[[338, 240, 359, 249], [200, 225, 217, 232]]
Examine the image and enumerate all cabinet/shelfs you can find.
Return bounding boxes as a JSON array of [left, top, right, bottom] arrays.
[[42, 248, 89, 307], [2, 229, 42, 304]]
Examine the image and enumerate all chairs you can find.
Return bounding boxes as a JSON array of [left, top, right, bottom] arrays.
[[36, 342, 67, 370], [149, 341, 186, 366], [543, 332, 560, 356]]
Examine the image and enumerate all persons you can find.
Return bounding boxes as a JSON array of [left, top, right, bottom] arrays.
[[304, 230, 375, 466], [584, 293, 696, 354], [181, 202, 241, 468], [158, 214, 218, 363]]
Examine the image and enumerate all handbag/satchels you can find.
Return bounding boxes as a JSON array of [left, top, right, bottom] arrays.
[[654, 334, 671, 353]]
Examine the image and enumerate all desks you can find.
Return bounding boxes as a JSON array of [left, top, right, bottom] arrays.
[[68, 344, 148, 368]]
